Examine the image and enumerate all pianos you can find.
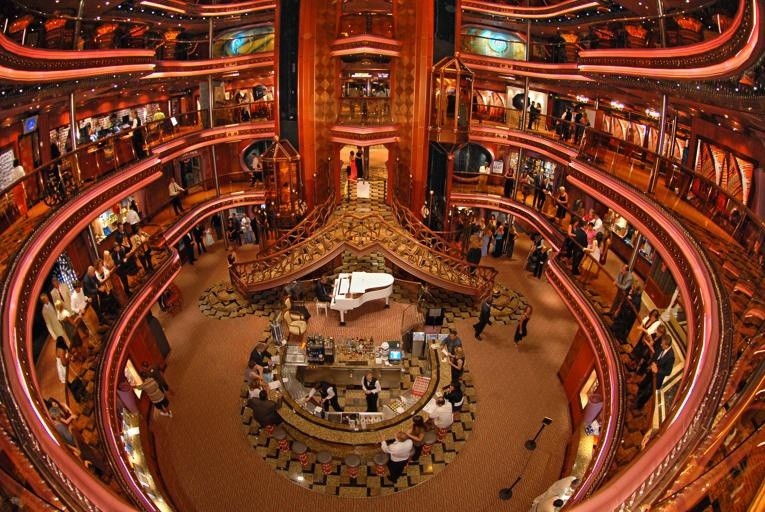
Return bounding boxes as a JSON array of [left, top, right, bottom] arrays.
[[330, 272, 395, 325]]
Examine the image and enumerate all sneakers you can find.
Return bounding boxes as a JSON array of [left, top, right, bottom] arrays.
[[160, 410, 172, 418]]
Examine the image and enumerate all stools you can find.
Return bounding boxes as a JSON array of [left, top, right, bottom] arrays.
[[316, 300, 328, 318], [265, 423, 448, 481]]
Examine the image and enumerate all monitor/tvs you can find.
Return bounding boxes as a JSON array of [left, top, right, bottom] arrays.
[[388, 349, 403, 361], [24, 115, 40, 134]]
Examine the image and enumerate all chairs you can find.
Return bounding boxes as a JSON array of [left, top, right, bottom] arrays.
[[285, 296, 307, 342]]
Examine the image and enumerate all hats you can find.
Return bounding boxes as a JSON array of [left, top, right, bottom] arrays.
[[454, 346, 463, 355]]
